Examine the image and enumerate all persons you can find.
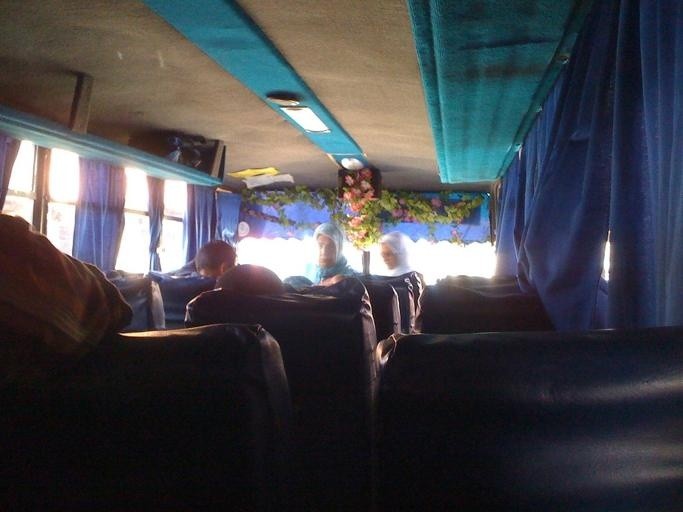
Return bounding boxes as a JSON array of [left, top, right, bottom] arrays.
[[283, 222, 356, 288], [214, 265, 283, 295], [0, 213, 133, 512], [378, 231, 415, 276], [195, 240, 236, 279]]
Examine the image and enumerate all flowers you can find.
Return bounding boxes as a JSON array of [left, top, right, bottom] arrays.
[[224, 157, 486, 254]]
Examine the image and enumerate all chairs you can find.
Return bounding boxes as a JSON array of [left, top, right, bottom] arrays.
[[0, 268, 683, 512]]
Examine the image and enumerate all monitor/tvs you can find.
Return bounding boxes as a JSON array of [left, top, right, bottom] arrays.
[[338, 169, 381, 200]]
